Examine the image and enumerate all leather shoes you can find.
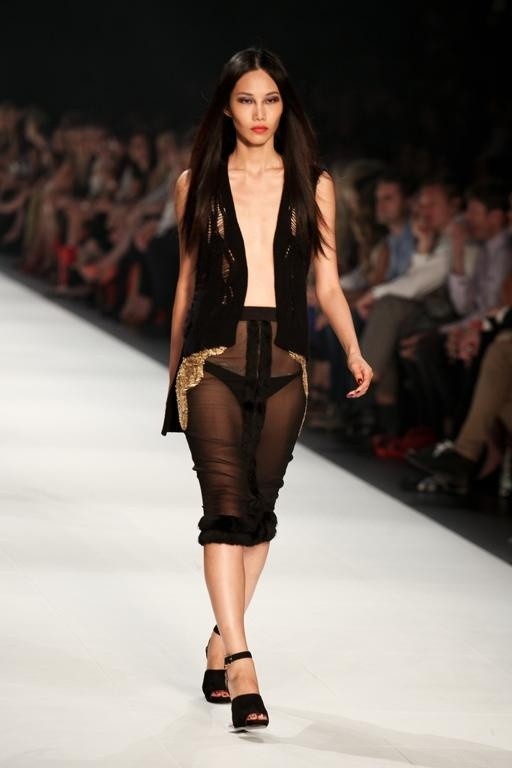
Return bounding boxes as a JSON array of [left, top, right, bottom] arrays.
[[309, 382, 489, 513]]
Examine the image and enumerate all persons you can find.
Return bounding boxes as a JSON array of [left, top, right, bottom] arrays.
[[298, 163, 511, 505], [2, 99, 205, 338], [161, 47, 374, 738]]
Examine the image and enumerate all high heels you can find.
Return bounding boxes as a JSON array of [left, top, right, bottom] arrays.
[[201, 624, 272, 732]]
